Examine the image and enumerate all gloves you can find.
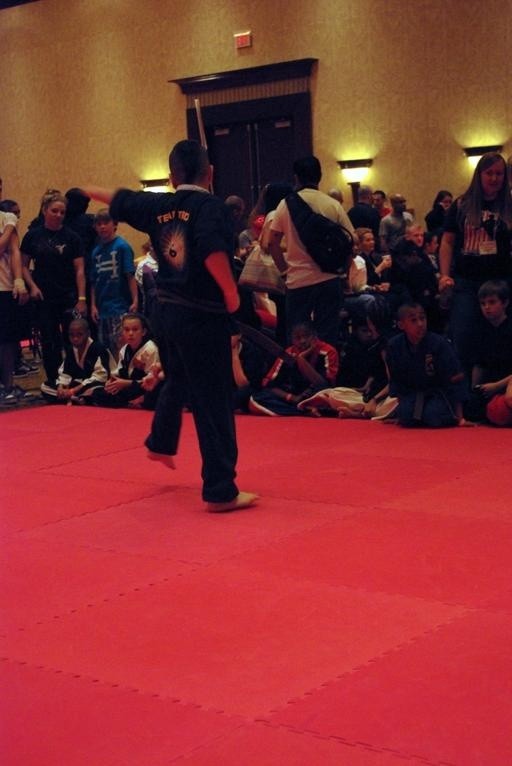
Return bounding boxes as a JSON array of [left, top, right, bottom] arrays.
[[11, 278, 31, 306]]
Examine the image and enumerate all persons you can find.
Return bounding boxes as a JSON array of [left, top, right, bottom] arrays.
[[263, 153, 359, 358], [75, 138, 261, 513], [436, 150, 510, 355], [0, 176, 510, 430]]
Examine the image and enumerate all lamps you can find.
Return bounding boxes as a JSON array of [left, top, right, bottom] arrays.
[[335, 159, 373, 183], [140, 179, 170, 192], [464, 144, 503, 169]]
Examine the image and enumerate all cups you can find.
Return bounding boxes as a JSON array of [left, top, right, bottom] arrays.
[[382, 254, 391, 265]]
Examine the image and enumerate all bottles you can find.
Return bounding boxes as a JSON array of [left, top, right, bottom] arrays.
[[438, 280, 453, 309]]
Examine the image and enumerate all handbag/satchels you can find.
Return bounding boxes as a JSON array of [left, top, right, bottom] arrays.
[[284, 190, 356, 276]]
